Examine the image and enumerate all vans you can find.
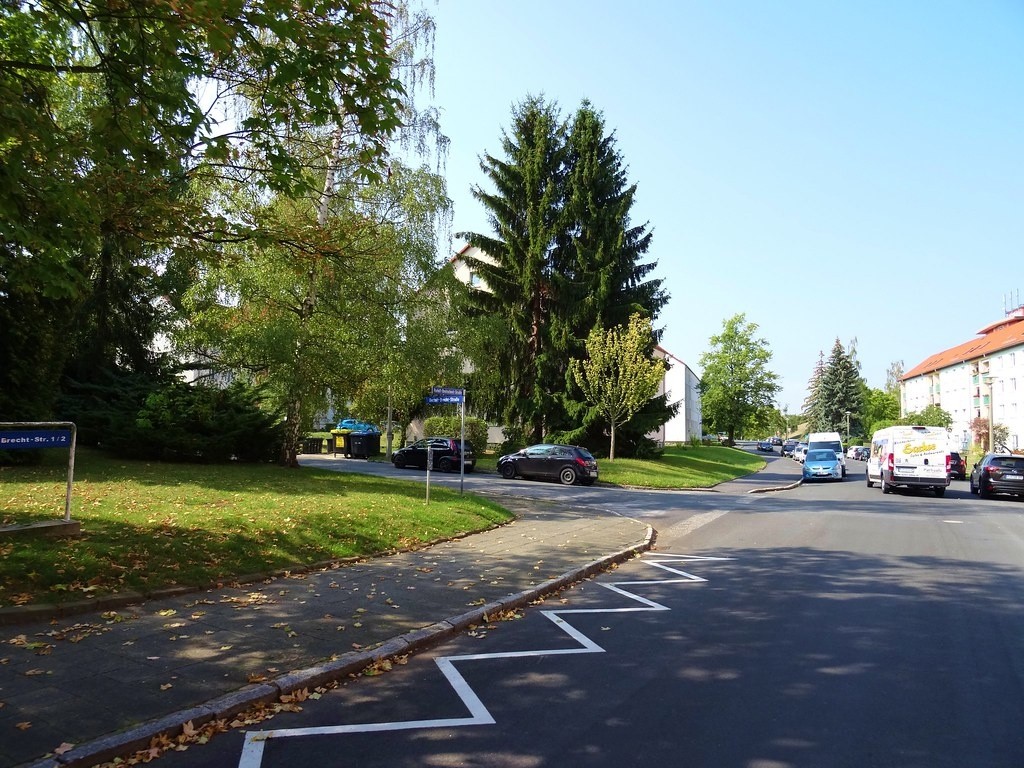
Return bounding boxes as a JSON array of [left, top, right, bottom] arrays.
[[380, 421, 402, 431]]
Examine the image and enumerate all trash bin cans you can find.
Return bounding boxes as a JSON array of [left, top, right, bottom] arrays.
[[331, 427, 382, 459]]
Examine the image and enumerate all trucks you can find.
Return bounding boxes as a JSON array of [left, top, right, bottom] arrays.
[[806, 432, 846, 477], [865, 425, 952, 496]]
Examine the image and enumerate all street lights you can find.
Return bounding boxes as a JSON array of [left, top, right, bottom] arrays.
[[984, 373, 999, 455], [845, 410, 851, 443]]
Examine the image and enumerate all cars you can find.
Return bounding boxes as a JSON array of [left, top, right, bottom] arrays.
[[391, 437, 476, 474], [802, 449, 843, 482], [756, 437, 807, 464], [337, 419, 380, 432], [496, 442, 598, 487], [951, 451, 968, 481], [970, 456, 1024, 499], [847, 446, 871, 462]]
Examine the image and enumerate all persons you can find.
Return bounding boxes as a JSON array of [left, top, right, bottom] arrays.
[[717, 434, 721, 442], [707, 434, 712, 441]]
